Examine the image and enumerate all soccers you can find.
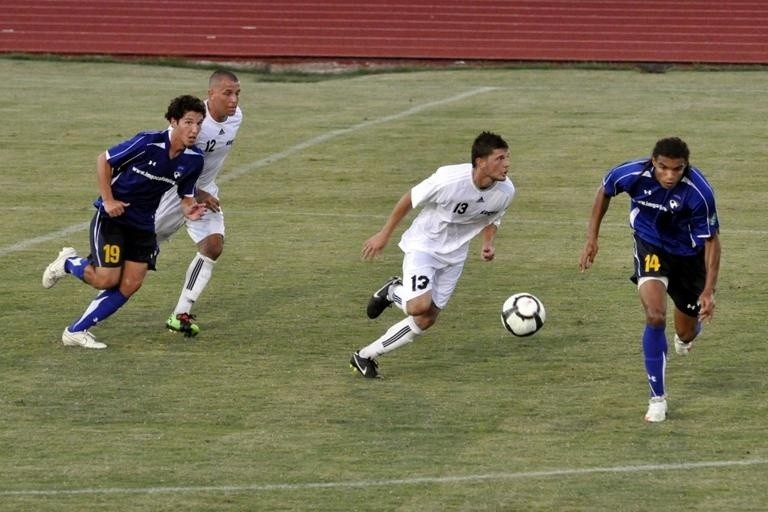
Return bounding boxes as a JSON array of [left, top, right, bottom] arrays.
[[501, 292, 546, 337]]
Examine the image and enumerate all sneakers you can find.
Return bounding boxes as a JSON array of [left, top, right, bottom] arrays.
[[643, 395, 669, 423], [166, 312, 200, 338], [41, 246, 78, 289], [366, 276, 402, 320], [349, 348, 385, 379], [62, 325, 108, 350], [673, 332, 693, 357]]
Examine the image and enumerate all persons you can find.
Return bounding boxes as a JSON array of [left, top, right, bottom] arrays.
[[42, 96, 206, 349], [579, 137, 721, 423], [153, 72, 242, 337], [350, 132, 515, 378]]
